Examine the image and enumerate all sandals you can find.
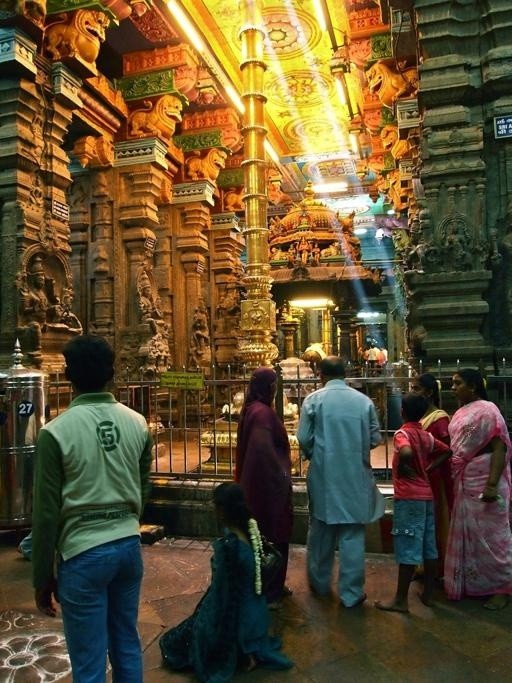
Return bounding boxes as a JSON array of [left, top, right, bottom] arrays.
[[483, 593, 508, 611]]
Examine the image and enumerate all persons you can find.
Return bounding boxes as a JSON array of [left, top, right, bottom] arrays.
[[233, 368, 299, 607], [444, 220, 467, 256], [355, 343, 390, 374], [157, 478, 295, 683], [137, 267, 172, 340], [409, 370, 451, 599], [291, 356, 383, 615], [23, 259, 66, 324], [375, 391, 455, 614], [446, 367, 512, 611], [30, 331, 155, 682]]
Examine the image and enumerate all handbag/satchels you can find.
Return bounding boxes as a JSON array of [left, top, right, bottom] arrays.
[[257, 546, 285, 596]]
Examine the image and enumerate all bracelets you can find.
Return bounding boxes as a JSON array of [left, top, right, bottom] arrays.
[[485, 484, 498, 492]]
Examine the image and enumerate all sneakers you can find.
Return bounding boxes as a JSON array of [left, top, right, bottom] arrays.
[[17, 543, 33, 560]]
